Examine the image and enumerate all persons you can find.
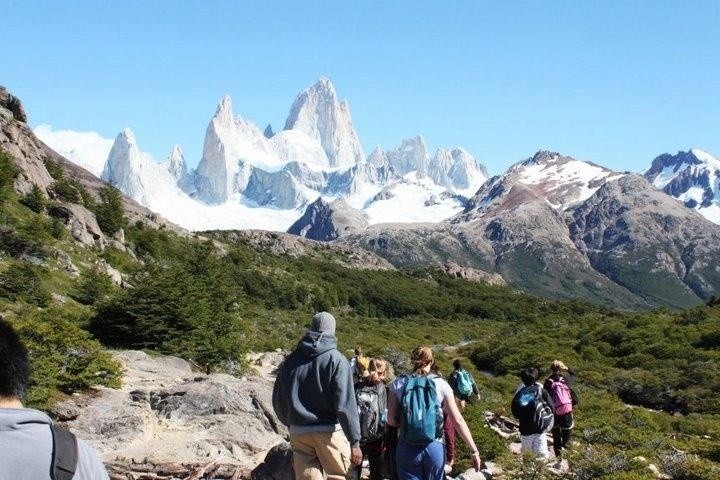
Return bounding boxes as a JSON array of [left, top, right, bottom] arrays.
[[346, 345, 482, 480], [272, 312, 363, 480], [510, 359, 579, 459], [0, 316, 111, 479]]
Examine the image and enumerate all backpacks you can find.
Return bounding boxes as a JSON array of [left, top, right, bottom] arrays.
[[547, 376, 574, 416], [354, 382, 388, 446], [528, 385, 555, 433], [400, 372, 445, 445], [455, 370, 473, 396]]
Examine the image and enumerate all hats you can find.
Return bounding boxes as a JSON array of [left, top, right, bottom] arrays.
[[310, 312, 336, 333]]
